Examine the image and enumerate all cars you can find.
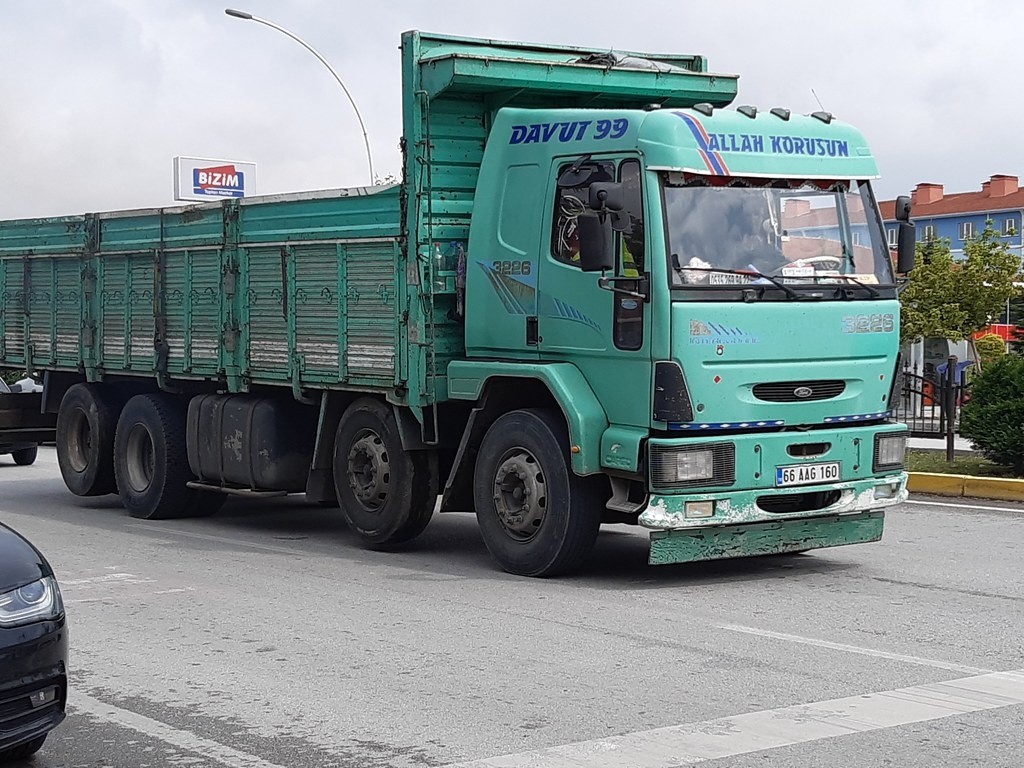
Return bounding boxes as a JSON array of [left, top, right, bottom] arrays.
[[1, 373, 43, 465], [0, 521, 68, 762]]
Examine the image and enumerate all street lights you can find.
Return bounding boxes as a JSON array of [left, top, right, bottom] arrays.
[[225, 9, 374, 185]]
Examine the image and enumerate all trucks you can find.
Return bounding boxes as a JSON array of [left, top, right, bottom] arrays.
[[0, 31, 914, 580]]
